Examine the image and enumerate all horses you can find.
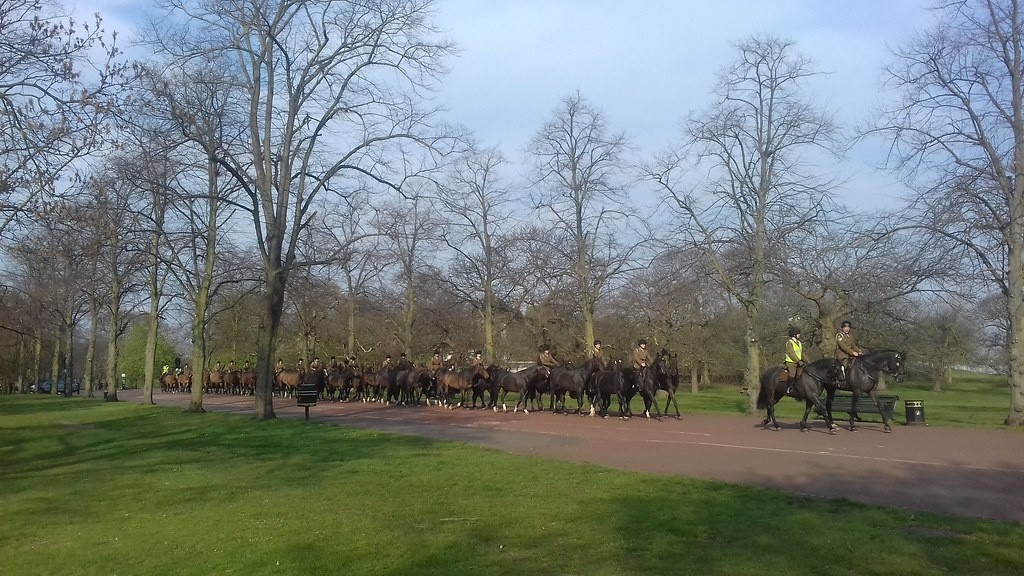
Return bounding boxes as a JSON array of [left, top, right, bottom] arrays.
[[159, 348, 682, 422], [824, 349, 908, 433], [756, 357, 847, 435]]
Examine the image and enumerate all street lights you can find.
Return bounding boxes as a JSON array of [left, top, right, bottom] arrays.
[[121, 374, 126, 389]]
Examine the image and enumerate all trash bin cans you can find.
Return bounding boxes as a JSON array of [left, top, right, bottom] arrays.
[[903, 400, 925, 426]]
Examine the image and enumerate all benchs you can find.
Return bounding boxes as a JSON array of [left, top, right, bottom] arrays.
[[817, 394, 899, 423]]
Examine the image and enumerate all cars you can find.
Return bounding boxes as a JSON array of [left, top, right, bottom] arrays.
[[29, 379, 79, 393]]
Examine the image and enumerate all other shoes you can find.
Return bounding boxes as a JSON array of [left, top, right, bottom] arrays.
[[787, 385, 792, 395]]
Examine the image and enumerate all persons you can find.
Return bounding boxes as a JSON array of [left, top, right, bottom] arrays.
[[588, 340, 607, 392], [472, 351, 484, 368], [632, 339, 654, 391], [430, 350, 442, 374], [835, 321, 863, 379], [537, 346, 560, 390], [159, 353, 410, 381], [784, 327, 810, 394]]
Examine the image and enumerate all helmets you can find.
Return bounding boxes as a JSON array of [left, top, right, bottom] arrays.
[[434, 349, 439, 354], [789, 327, 800, 337], [841, 321, 852, 328], [164, 360, 249, 369], [386, 355, 391, 359], [331, 356, 335, 359], [638, 339, 647, 345], [401, 353, 406, 358], [279, 359, 283, 363], [542, 344, 550, 351], [314, 356, 319, 359], [594, 340, 601, 346], [350, 356, 356, 360], [299, 358, 303, 362], [476, 350, 482, 354]]
